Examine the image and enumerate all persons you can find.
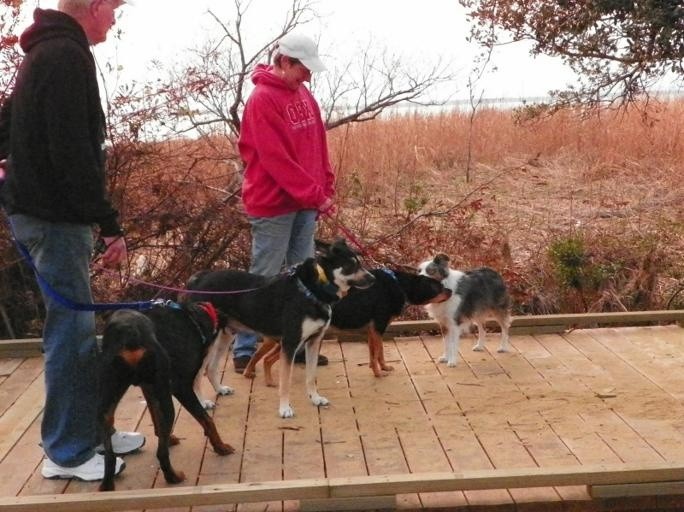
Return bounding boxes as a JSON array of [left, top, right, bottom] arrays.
[[226, 33, 334, 375], [3, 1, 147, 481]]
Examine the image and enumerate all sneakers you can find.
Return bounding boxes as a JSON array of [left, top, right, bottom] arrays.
[[39, 443, 126, 483], [93, 431, 146, 454], [233, 355, 256, 373], [287, 349, 329, 365]]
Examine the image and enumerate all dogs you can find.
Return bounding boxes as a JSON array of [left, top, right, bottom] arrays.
[[96, 301, 235, 491], [176, 238, 376, 418], [243, 269, 452, 388], [416, 253, 515, 368]]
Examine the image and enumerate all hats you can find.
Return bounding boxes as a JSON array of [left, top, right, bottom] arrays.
[[278, 30, 328, 72]]
